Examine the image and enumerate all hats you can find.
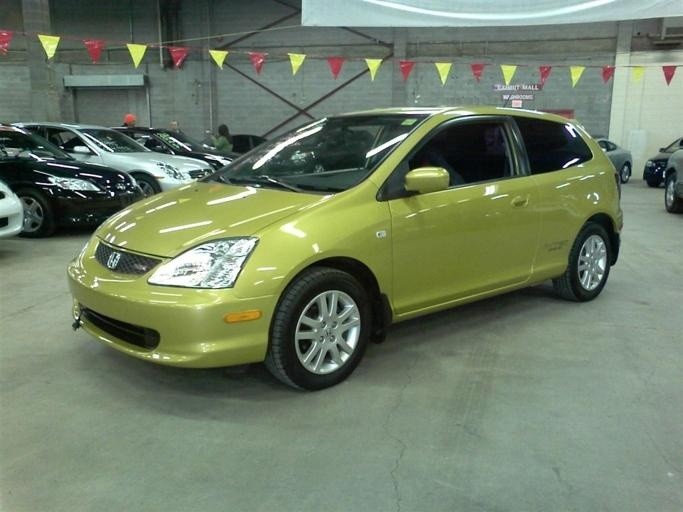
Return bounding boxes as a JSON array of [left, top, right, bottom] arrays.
[[125, 115, 136, 122]]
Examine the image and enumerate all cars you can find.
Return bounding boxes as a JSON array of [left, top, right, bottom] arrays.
[[664, 150, 682, 213], [64, 127, 244, 172], [263, 129, 373, 174], [593, 137, 633, 183], [65, 105, 625, 393], [0, 124, 135, 237], [0, 181, 24, 239], [10, 123, 217, 199], [642, 136, 683, 188]]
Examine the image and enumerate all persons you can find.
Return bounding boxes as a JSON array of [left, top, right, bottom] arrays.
[[209, 125, 234, 154], [119, 114, 142, 147]]
